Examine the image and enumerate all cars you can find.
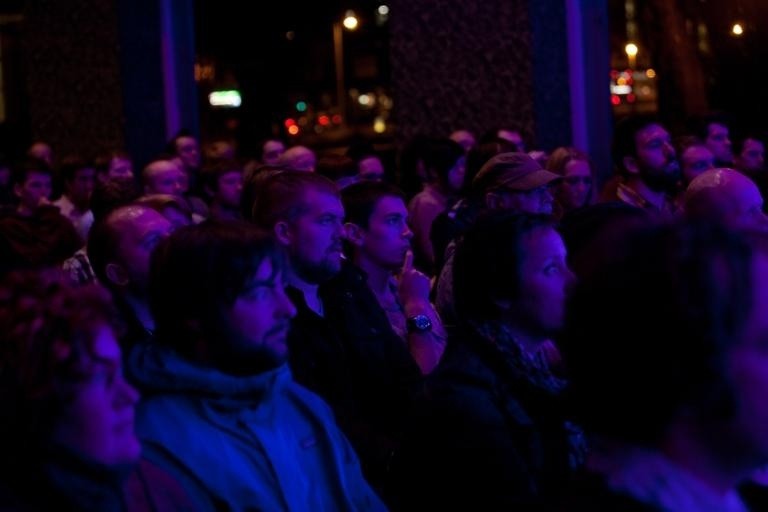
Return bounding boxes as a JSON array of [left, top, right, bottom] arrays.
[[607, 66, 659, 107]]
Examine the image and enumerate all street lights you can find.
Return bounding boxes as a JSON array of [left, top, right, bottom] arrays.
[[731, 22, 744, 99], [377, 6, 390, 29], [333, 11, 360, 112], [624, 42, 639, 69]]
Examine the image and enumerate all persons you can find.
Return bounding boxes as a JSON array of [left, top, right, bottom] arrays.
[[2, 107, 768, 512]]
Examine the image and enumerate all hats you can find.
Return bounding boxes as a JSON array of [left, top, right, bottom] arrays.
[[474, 152, 563, 191]]
